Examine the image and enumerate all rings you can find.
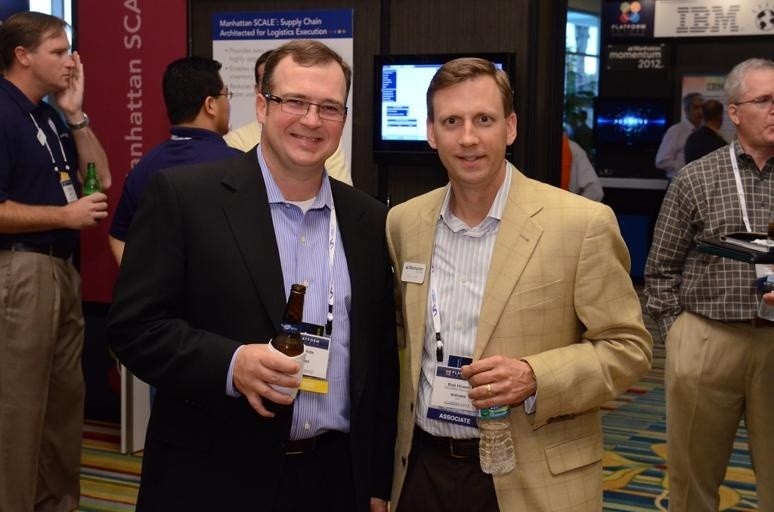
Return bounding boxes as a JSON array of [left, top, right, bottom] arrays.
[[487, 384, 492, 397]]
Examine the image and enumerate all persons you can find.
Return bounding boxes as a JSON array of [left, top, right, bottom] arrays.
[[655, 93, 705, 189], [109, 57, 246, 269], [386, 57, 654, 512], [684, 99, 730, 165], [567, 113, 578, 135], [106, 40, 398, 512], [569, 110, 593, 151], [223, 49, 354, 187], [560, 133, 604, 202]]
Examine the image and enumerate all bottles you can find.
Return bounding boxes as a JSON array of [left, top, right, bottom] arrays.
[[82, 162, 101, 221], [475, 401, 517, 476], [262, 283, 305, 415]]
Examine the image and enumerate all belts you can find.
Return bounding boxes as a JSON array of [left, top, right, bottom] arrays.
[[282, 431, 346, 468], [415, 429, 482, 460], [0, 240, 71, 260], [718, 317, 773, 328]]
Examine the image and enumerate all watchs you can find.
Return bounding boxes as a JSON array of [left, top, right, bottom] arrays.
[[0, 13, 112, 512], [67, 112, 89, 130]]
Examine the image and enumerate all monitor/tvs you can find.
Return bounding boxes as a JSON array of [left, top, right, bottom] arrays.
[[590, 96, 681, 149], [374, 50, 516, 156]]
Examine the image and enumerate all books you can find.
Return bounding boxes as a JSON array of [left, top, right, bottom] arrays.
[[643, 57, 774, 512], [695, 240, 774, 264], [719, 233, 774, 253]]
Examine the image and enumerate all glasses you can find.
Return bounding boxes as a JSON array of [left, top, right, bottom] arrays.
[[264, 94, 347, 122], [733, 94, 774, 109], [210, 91, 232, 100]]
